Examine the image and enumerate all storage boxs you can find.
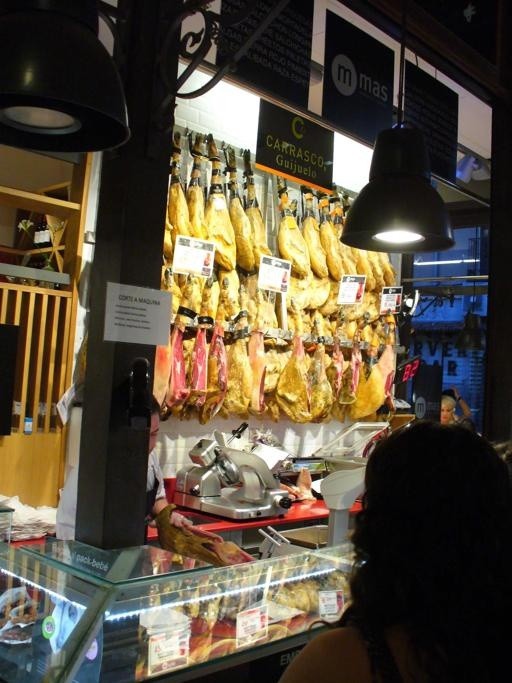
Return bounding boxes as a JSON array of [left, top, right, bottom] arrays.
[[322, 455, 370, 502]]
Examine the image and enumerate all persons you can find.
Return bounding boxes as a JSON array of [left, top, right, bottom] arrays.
[[440, 388, 471, 424], [144, 395, 193, 544], [278, 422, 512, 683]]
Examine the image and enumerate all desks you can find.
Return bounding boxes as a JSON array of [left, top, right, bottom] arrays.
[[144, 495, 362, 563]]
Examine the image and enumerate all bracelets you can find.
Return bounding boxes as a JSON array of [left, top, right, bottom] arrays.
[[457, 396, 462, 401]]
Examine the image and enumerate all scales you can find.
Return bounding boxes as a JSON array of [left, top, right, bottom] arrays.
[[258, 466, 367, 561]]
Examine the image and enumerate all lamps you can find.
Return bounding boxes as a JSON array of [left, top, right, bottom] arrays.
[[339, 0, 455, 255], [450, 151, 482, 182], [0, 0, 141, 155]]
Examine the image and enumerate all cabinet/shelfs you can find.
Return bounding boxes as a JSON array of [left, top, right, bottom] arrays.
[[0, 511, 362, 682]]
